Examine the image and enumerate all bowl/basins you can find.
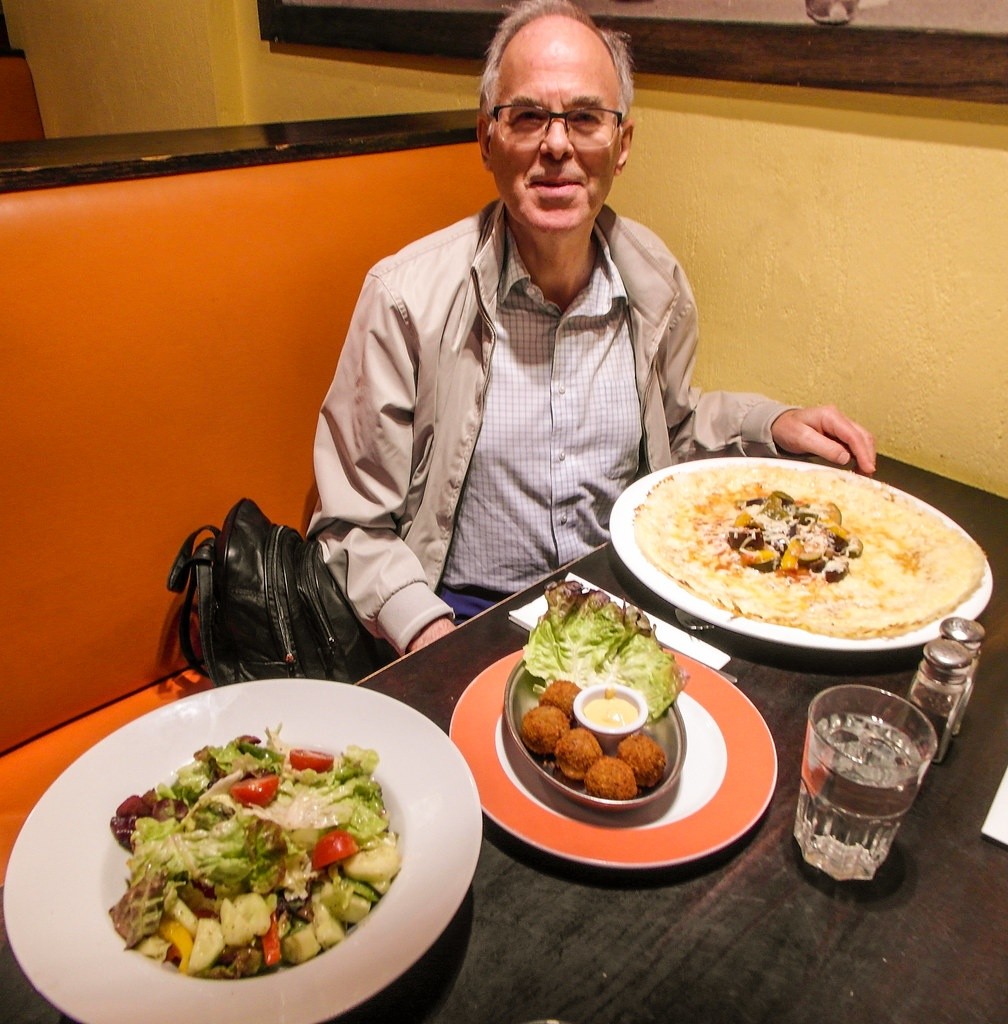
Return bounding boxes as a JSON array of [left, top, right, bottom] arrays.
[[504, 656, 688, 815]]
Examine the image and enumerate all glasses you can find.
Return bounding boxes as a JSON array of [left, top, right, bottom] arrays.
[[490, 104, 625, 150]]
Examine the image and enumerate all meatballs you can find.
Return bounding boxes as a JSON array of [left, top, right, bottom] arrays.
[[520, 679, 669, 801]]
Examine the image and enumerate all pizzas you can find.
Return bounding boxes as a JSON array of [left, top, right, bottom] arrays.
[[633, 466, 985, 636]]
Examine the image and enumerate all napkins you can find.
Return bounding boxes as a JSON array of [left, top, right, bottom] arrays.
[[508, 571, 731, 671]]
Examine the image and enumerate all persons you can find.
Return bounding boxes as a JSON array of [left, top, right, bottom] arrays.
[[306, 0, 877, 658]]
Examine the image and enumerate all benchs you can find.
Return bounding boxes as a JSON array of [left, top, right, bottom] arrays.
[[0, 109, 500, 886]]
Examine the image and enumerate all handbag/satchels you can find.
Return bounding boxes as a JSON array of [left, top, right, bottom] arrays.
[[166, 498, 378, 687]]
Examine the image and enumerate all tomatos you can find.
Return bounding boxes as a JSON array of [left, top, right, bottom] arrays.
[[287, 749, 334, 772], [229, 774, 279, 808], [312, 831, 358, 867]]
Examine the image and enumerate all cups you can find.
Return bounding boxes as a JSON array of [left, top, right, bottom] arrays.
[[792, 683, 939, 881]]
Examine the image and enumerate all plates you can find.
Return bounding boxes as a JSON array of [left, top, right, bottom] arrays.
[[610, 456, 994, 651], [2, 677, 484, 1024], [448, 646, 779, 871]]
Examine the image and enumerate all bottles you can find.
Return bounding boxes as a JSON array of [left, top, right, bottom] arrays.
[[933, 617, 986, 737], [896, 638, 973, 764]]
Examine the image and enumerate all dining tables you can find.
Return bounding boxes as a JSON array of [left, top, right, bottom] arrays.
[[0, 450, 1008, 1024]]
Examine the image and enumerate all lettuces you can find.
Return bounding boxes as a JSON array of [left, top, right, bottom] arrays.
[[107, 723, 400, 978], [521, 579, 690, 725]]
